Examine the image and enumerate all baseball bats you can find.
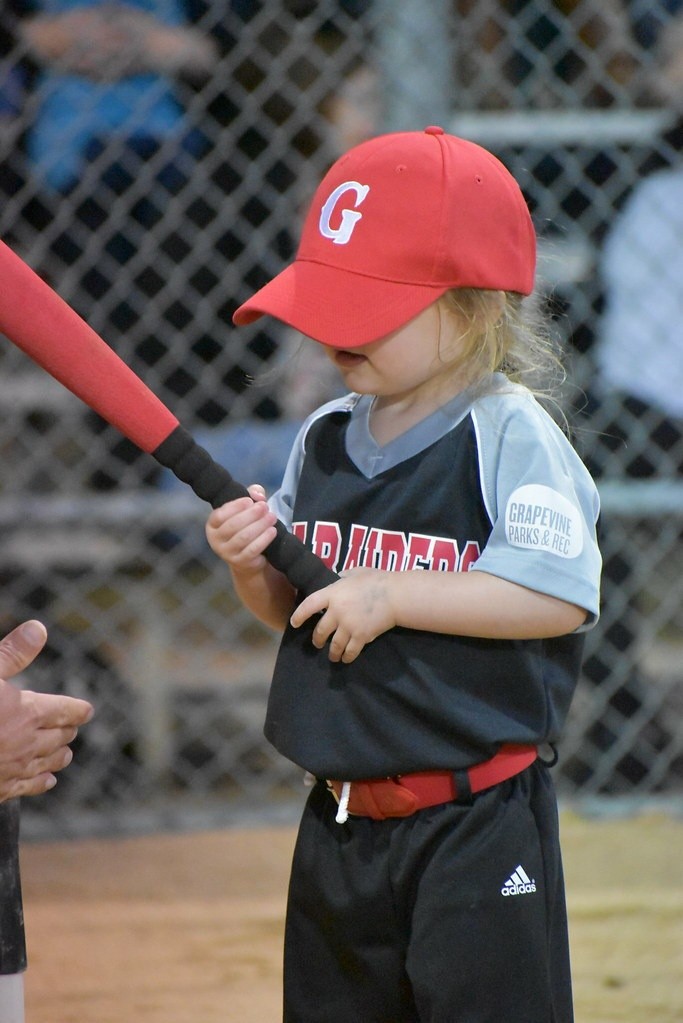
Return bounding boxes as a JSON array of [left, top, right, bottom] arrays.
[[1, 237, 404, 676]]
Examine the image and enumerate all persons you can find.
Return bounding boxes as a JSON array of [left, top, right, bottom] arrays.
[[547, 117, 683, 799], [204, 128, 606, 1023], [0, 618, 95, 1023], [0, 0, 219, 487]]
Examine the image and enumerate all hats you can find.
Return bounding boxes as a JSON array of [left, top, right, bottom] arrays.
[[232, 126, 536, 349]]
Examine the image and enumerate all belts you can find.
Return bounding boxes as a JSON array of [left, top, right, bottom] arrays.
[[326, 743, 539, 824]]
